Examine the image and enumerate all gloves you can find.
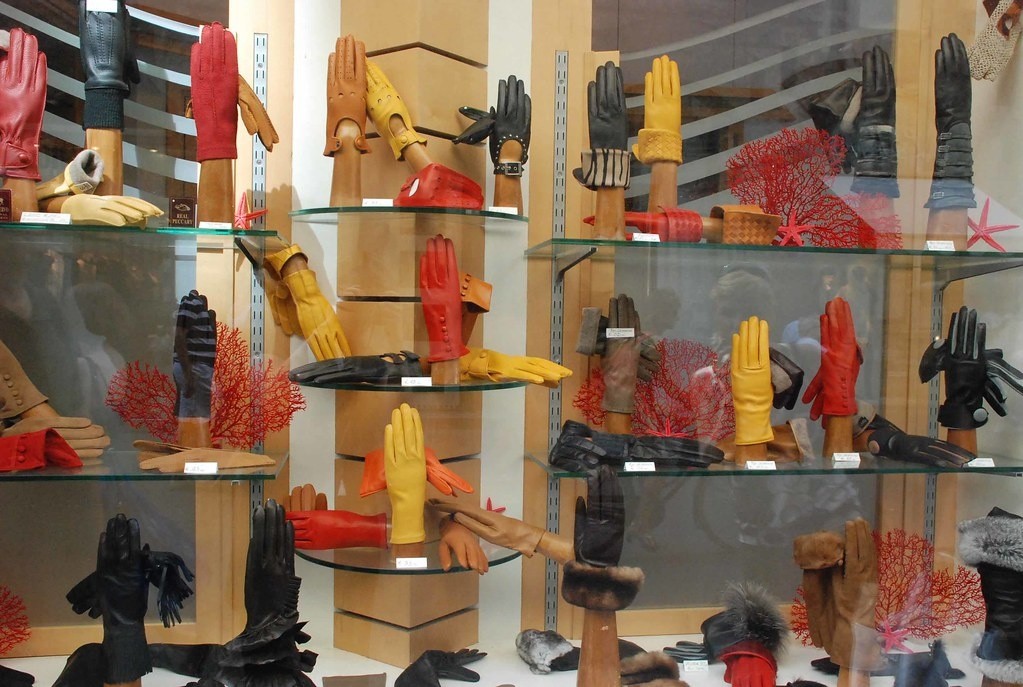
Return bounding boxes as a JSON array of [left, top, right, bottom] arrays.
[[282, 402, 581, 687], [0, 288, 277, 474], [265, 234, 574, 389], [548, 292, 1023, 472], [323, 33, 533, 210], [561, 464, 1023, 687], [0, 0, 279, 231], [0, 497, 320, 687], [572, 31, 978, 210]]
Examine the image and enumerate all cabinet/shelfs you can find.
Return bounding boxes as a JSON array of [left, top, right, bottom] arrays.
[[1, 33, 1023, 687]]
